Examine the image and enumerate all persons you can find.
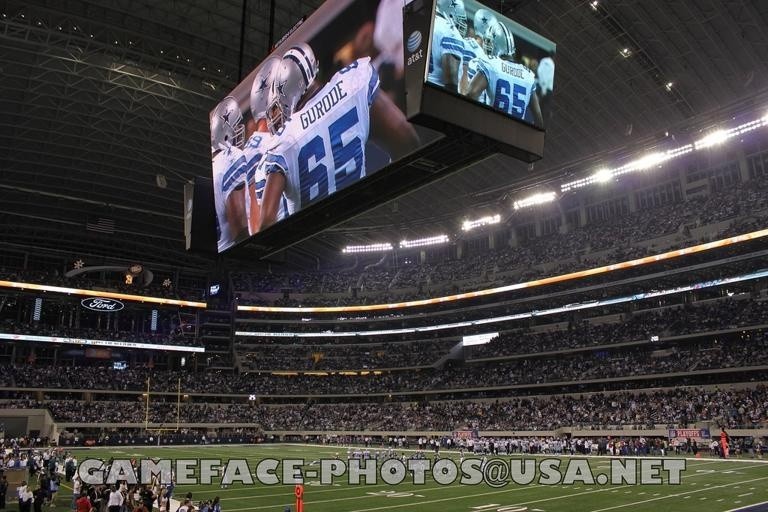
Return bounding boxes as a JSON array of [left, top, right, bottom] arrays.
[[0, 444, 223, 512], [1, 170, 767, 462], [427, 1, 555, 130], [209, 42, 425, 252]]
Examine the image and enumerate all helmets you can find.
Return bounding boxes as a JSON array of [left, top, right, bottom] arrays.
[[484, 22, 515, 60], [437, 0, 468, 39], [474, 8, 497, 39], [249, 54, 282, 124], [276, 41, 318, 118], [209, 96, 246, 152]]
